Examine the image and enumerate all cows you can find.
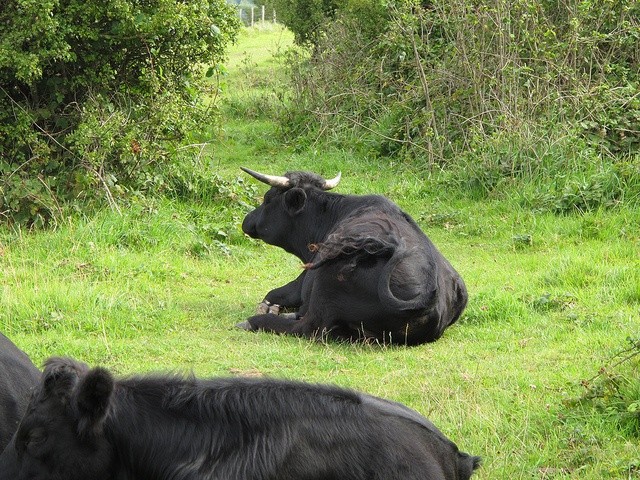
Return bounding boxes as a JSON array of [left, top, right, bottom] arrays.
[[0, 355, 482, 479], [235, 166, 468, 347]]
